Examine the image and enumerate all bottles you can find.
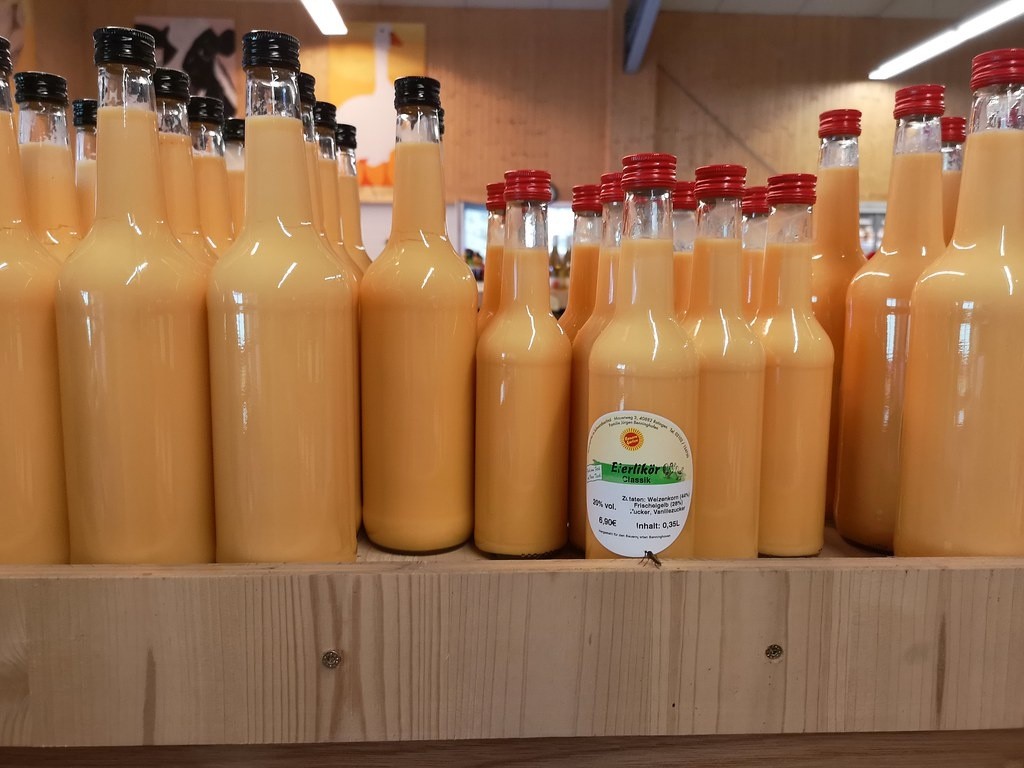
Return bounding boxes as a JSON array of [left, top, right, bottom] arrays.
[[0, 23, 1024, 564]]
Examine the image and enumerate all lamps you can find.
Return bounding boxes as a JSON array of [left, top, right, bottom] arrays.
[[300, 0, 348, 36], [868, 0, 1024, 81]]
